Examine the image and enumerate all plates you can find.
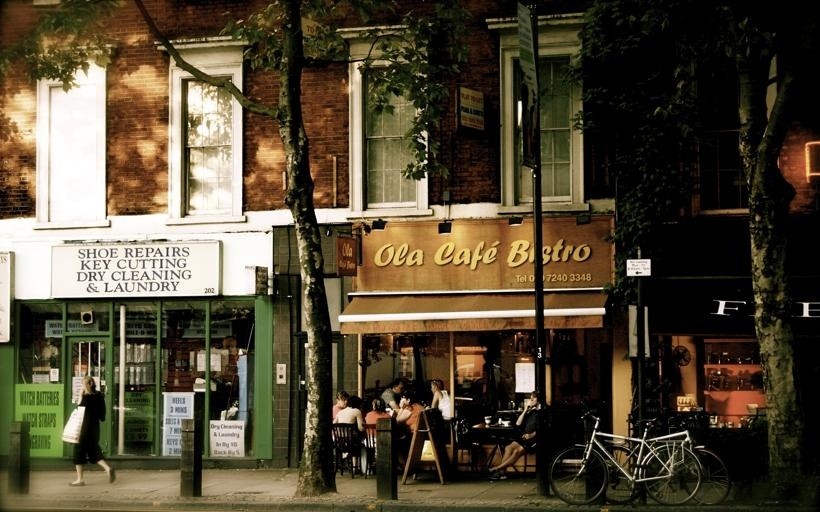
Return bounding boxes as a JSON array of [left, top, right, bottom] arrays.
[[502, 425, 512, 427]]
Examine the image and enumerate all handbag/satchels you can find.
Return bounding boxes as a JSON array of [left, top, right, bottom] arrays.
[[61, 406, 86, 444], [524, 409, 544, 433]]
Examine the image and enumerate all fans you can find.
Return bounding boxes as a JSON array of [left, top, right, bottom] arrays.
[[675, 345, 692, 367]]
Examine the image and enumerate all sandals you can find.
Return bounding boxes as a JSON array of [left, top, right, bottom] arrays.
[[69, 481, 85, 486], [107, 467, 115, 483]]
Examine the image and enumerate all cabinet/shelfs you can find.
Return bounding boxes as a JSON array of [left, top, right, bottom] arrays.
[[703, 337, 772, 424]]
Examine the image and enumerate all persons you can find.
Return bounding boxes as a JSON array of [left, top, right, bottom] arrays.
[[488, 391, 541, 480], [332, 376, 453, 473], [69, 377, 116, 487]]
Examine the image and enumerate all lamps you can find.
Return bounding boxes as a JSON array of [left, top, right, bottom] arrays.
[[437, 220, 454, 234], [371, 218, 388, 231], [508, 218, 523, 227]]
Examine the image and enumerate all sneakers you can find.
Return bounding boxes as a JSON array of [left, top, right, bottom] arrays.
[[353, 466, 363, 475], [488, 467, 507, 480]]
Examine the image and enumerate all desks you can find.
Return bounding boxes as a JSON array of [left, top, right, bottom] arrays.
[[472, 421, 529, 476]]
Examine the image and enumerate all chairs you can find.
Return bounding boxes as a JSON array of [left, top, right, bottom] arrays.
[[449, 416, 486, 479], [333, 421, 378, 479]]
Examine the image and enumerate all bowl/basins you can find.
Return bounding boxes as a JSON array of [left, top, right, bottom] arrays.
[[502, 421, 511, 425]]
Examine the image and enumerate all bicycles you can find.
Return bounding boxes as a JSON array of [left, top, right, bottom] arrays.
[[550, 409, 731, 506]]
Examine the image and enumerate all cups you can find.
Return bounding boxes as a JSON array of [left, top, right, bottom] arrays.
[[485, 416, 492, 424]]
